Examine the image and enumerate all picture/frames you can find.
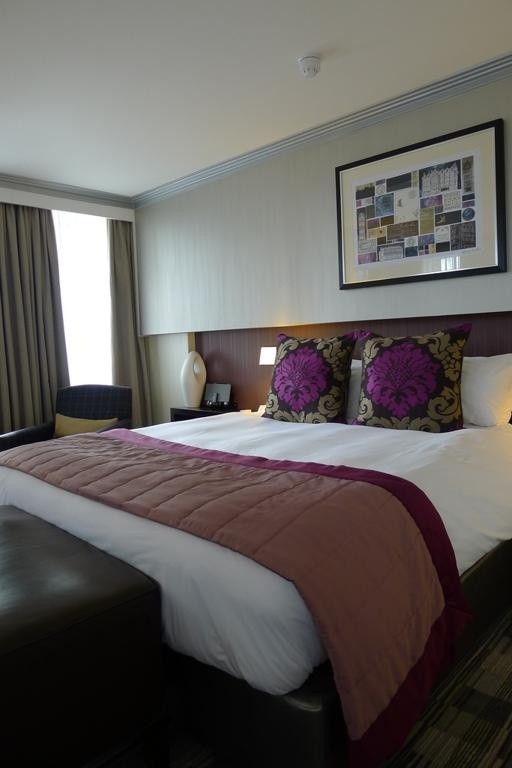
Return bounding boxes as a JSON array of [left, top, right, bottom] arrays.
[[334, 117, 507, 291]]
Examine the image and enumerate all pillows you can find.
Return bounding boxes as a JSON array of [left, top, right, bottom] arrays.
[[260, 323, 512, 433], [53, 412, 119, 439]]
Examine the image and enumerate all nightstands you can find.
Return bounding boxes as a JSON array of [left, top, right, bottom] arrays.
[[169, 407, 227, 421]]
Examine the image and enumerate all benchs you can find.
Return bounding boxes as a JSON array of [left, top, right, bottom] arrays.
[[0, 503, 163, 766]]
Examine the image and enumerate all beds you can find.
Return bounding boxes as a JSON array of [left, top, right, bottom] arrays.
[[0, 411, 511, 767]]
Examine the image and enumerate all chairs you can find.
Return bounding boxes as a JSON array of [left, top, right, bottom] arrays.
[[1, 384, 133, 454]]
